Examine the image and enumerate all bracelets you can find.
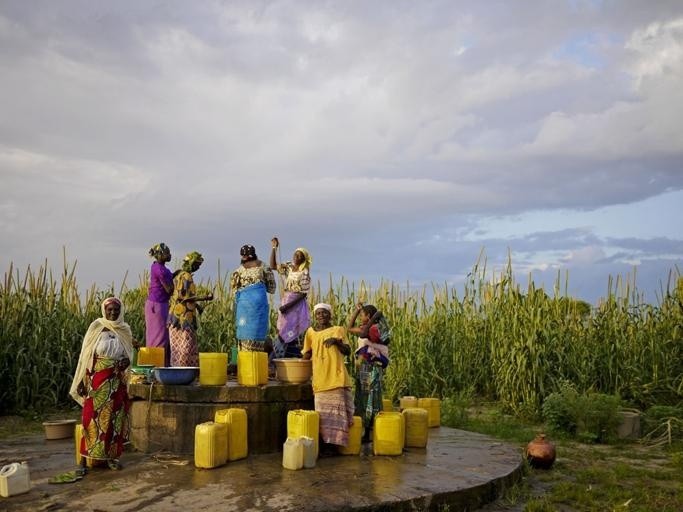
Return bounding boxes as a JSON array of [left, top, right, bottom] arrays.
[[271, 246, 277, 250]]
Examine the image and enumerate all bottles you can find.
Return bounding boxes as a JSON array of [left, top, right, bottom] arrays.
[[525, 439, 556, 468]]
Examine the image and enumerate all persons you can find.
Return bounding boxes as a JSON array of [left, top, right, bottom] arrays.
[[347, 300, 390, 446], [300, 302, 356, 455], [228, 243, 278, 353], [140, 241, 182, 366], [68, 296, 135, 477], [268, 236, 314, 376], [163, 250, 214, 369]]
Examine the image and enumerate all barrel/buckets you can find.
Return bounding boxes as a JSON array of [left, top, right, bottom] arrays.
[[372, 406, 429, 454], [397, 395, 442, 427], [339, 416, 362, 455], [383, 398, 393, 411], [283, 409, 319, 470], [198, 352, 229, 386], [194, 407, 249, 468], [135, 347, 166, 368], [236, 349, 269, 388], [0, 460, 32, 498]]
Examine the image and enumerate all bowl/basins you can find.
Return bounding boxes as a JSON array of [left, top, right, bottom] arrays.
[[41, 419, 76, 440], [272, 357, 311, 383], [152, 366, 198, 385]]
